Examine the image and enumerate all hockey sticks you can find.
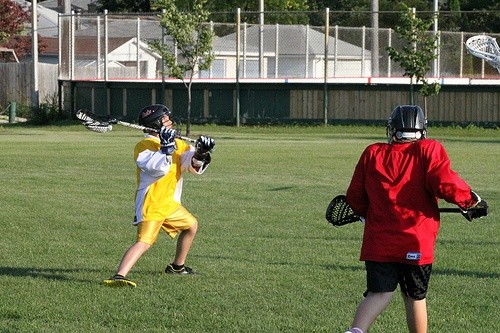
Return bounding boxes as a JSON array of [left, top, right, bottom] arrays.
[[324, 194, 462, 227], [76, 108, 216, 154], [465, 33, 500, 74]]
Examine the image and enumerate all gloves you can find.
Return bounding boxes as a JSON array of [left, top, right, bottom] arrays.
[[459, 190, 489, 222], [158, 125, 178, 155], [193, 135, 217, 162]]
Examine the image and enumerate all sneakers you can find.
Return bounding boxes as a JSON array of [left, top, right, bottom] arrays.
[[101, 275, 137, 289], [164, 263, 194, 275]]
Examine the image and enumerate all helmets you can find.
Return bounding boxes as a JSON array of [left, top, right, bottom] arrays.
[[138, 104, 172, 130], [386, 104, 428, 145]]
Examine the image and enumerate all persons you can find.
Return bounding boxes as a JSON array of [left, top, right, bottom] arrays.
[[345, 104, 488, 333], [102, 105, 215, 288]]
